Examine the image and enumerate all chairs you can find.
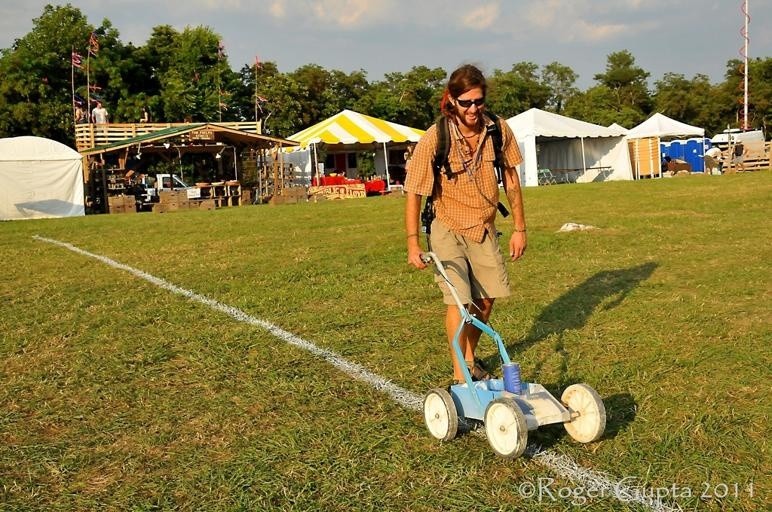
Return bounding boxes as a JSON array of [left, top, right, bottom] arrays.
[[538, 169, 557, 186]]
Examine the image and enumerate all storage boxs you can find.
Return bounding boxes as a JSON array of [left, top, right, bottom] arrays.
[[271, 187, 306, 205], [153, 189, 216, 213], [108, 195, 136, 213]]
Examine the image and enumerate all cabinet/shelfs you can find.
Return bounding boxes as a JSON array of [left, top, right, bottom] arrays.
[[107, 169, 126, 190]]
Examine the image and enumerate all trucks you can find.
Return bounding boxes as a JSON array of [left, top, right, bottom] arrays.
[[709, 128, 767, 168], [137, 170, 189, 204]]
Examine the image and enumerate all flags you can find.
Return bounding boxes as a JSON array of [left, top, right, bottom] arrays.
[[218, 43, 226, 60], [71, 51, 86, 70], [90, 33, 100, 57], [256, 56, 263, 69]]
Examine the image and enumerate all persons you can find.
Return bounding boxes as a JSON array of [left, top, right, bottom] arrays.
[[402, 63, 528, 384], [733, 139, 746, 173], [75, 103, 90, 148], [140, 105, 152, 132], [91, 101, 109, 142]]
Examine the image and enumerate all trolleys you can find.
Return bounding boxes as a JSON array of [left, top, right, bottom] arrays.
[[409, 249, 609, 459]]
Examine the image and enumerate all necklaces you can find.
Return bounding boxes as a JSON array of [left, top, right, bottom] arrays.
[[462, 132, 481, 138]]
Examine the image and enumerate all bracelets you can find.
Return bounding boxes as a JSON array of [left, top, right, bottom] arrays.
[[407, 234, 419, 239], [514, 229, 526, 232]]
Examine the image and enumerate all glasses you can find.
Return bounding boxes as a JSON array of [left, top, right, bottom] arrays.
[[456, 96, 486, 108]]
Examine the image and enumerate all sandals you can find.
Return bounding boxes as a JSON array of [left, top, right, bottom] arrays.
[[465, 360, 498, 381]]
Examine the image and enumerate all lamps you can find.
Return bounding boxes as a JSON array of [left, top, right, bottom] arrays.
[[215, 146, 226, 158], [164, 143, 170, 150], [136, 143, 142, 160]]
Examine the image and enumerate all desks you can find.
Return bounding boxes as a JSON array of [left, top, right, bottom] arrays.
[[366, 181, 386, 196]]
